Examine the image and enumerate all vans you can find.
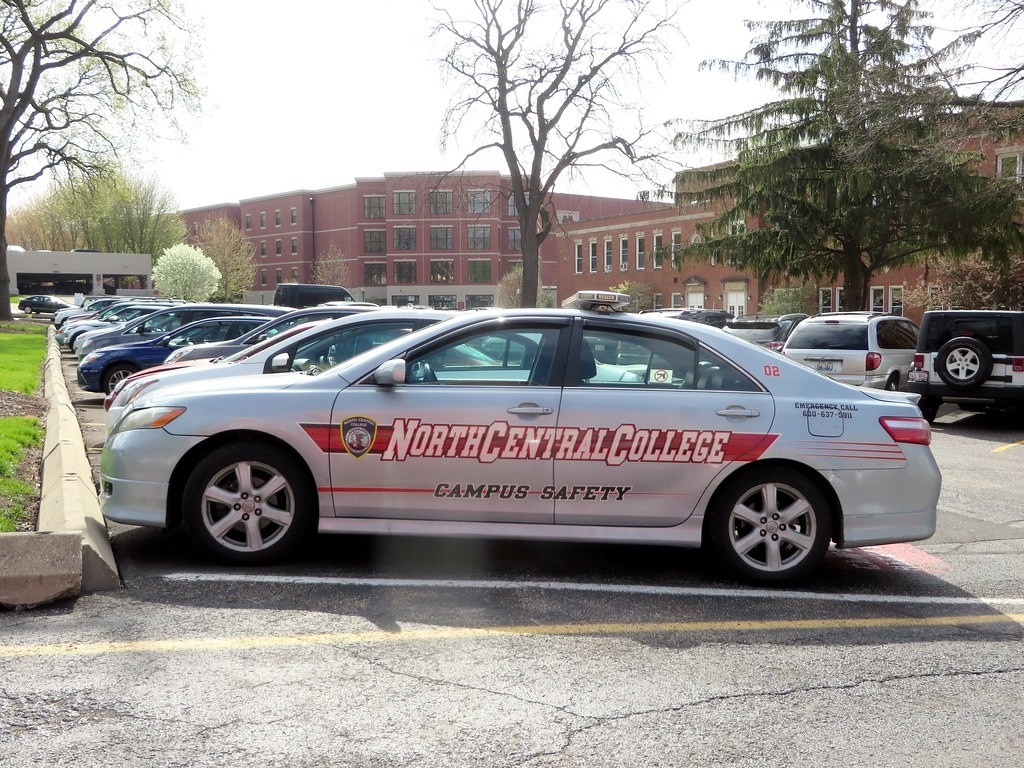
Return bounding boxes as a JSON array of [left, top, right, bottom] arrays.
[[272, 283, 357, 308]]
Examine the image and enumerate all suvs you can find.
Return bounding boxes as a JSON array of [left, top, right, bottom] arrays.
[[778, 310, 941, 424], [907, 310, 1024, 427]]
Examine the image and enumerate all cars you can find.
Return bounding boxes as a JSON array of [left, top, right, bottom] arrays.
[[18, 295, 82, 314], [50, 289, 941, 586]]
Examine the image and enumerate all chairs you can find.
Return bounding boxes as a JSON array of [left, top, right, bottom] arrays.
[[570, 338, 597, 384]]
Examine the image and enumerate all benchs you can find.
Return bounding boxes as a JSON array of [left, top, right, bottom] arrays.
[[678, 360, 731, 389]]
